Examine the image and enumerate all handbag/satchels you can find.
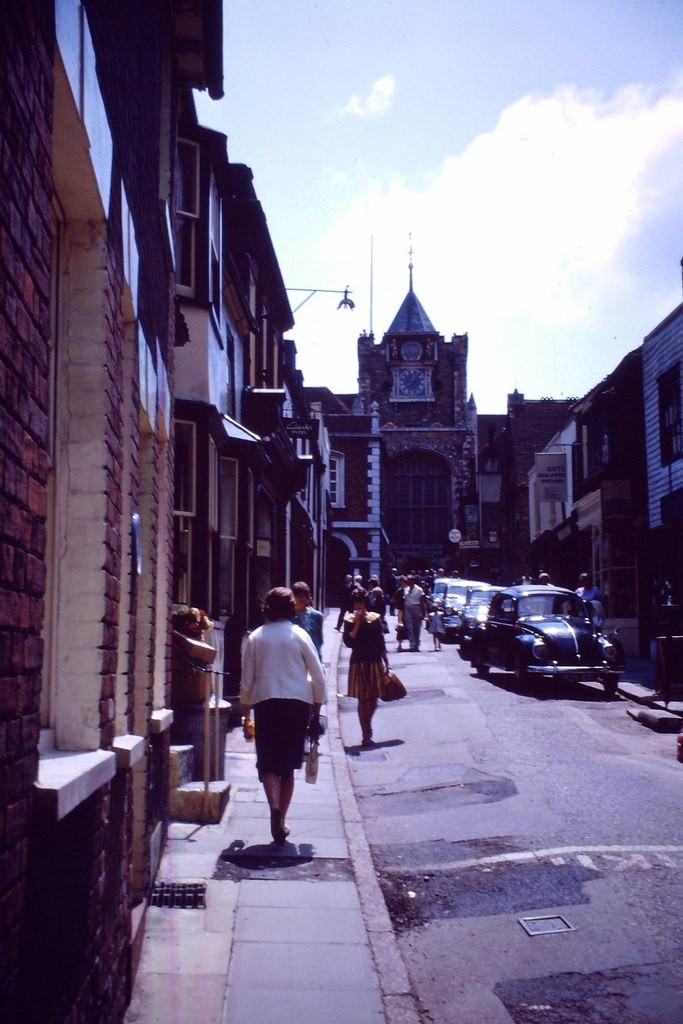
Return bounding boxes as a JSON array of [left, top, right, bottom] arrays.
[[395, 620, 409, 640], [380, 667, 407, 701], [305, 731, 317, 783]]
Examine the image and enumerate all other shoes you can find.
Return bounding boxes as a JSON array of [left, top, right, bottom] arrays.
[[274, 826, 290, 846], [271, 808, 281, 839], [363, 729, 372, 748]]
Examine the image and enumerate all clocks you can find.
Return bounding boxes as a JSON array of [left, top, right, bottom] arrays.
[[388, 366, 435, 402]]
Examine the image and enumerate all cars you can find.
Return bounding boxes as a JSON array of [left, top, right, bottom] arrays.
[[425, 577, 508, 636], [456, 585, 625, 694]]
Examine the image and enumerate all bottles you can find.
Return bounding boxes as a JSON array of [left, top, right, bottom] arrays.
[[245, 717, 254, 743], [304, 742, 320, 785]]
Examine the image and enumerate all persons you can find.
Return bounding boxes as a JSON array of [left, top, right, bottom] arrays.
[[514, 565, 554, 587], [239, 586, 327, 845], [576, 573, 604, 637], [334, 567, 462, 746], [290, 582, 324, 663]]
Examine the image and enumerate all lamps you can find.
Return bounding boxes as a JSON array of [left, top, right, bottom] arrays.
[[285, 284, 354, 313]]
